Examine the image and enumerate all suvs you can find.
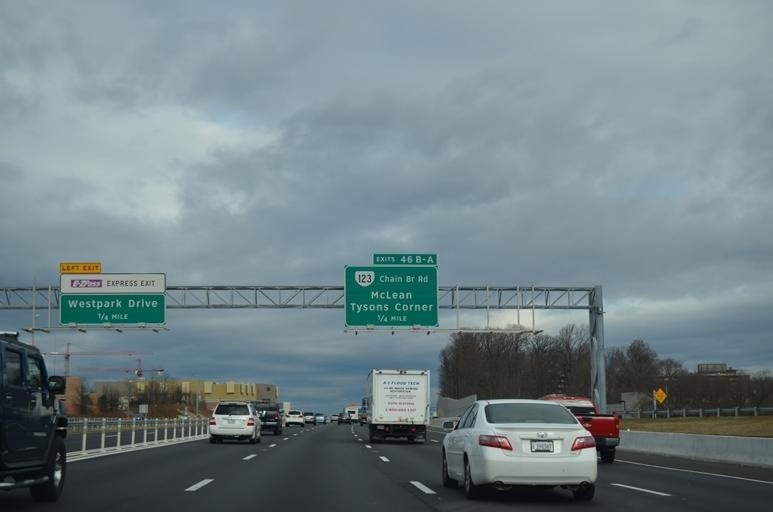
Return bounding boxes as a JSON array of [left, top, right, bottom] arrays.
[[0, 330, 71, 504]]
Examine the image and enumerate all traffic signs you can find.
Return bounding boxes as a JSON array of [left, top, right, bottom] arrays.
[[373, 251, 438, 266], [59, 274, 167, 295], [56, 292, 167, 328], [344, 264, 440, 331]]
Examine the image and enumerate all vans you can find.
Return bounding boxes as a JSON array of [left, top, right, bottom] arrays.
[[134, 417, 145, 424]]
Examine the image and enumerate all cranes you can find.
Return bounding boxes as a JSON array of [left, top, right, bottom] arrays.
[[78, 356, 164, 380], [38, 343, 153, 375]]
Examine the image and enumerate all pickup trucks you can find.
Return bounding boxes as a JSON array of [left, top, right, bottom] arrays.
[[537, 394, 622, 464]]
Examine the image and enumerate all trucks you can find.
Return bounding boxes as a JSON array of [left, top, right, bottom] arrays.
[[364, 367, 431, 444]]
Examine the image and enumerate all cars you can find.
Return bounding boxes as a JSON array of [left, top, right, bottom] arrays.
[[208, 397, 369, 444], [441, 397, 599, 505]]
